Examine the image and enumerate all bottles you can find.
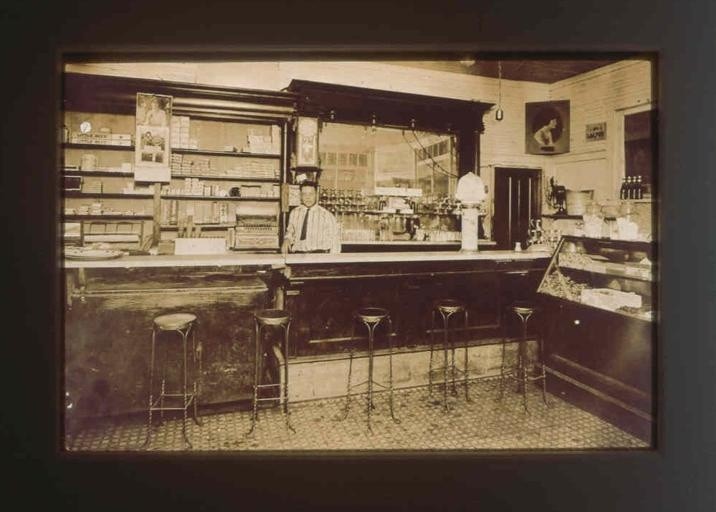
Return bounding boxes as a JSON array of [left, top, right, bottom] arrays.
[[621, 175, 643, 200], [320, 187, 364, 205]]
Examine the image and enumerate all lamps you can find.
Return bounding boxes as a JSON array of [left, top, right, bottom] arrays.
[[456, 171, 488, 252]]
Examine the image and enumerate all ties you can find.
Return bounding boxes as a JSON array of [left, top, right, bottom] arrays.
[[300, 209, 310, 240]]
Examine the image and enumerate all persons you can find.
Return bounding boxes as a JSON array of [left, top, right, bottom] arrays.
[[534, 113, 560, 146], [280, 179, 345, 255], [144, 98, 169, 125], [137, 97, 149, 125]]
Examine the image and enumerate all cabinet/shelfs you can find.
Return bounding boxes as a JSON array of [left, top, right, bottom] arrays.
[[536, 234, 656, 445], [63, 86, 293, 257]]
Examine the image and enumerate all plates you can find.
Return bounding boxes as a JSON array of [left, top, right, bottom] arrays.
[[64, 250, 122, 260]]
